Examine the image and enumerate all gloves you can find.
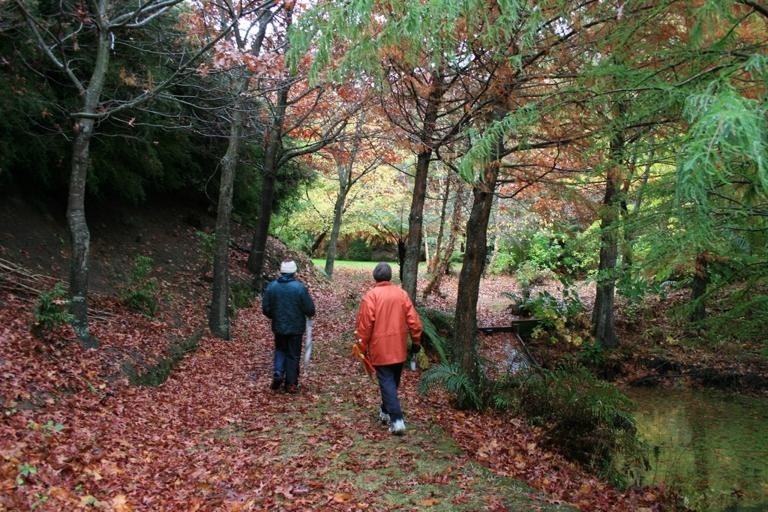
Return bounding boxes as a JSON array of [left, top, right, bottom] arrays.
[[412, 343, 420, 354]]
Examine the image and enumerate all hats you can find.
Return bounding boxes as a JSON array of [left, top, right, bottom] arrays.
[[280, 258, 297, 274], [373, 263, 391, 282]]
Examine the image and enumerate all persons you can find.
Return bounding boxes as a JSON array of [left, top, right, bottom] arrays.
[[262, 258, 316, 394], [354, 262, 424, 434]]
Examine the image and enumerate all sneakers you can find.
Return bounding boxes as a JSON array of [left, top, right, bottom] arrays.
[[380, 408, 407, 434], [270, 380, 298, 393]]
